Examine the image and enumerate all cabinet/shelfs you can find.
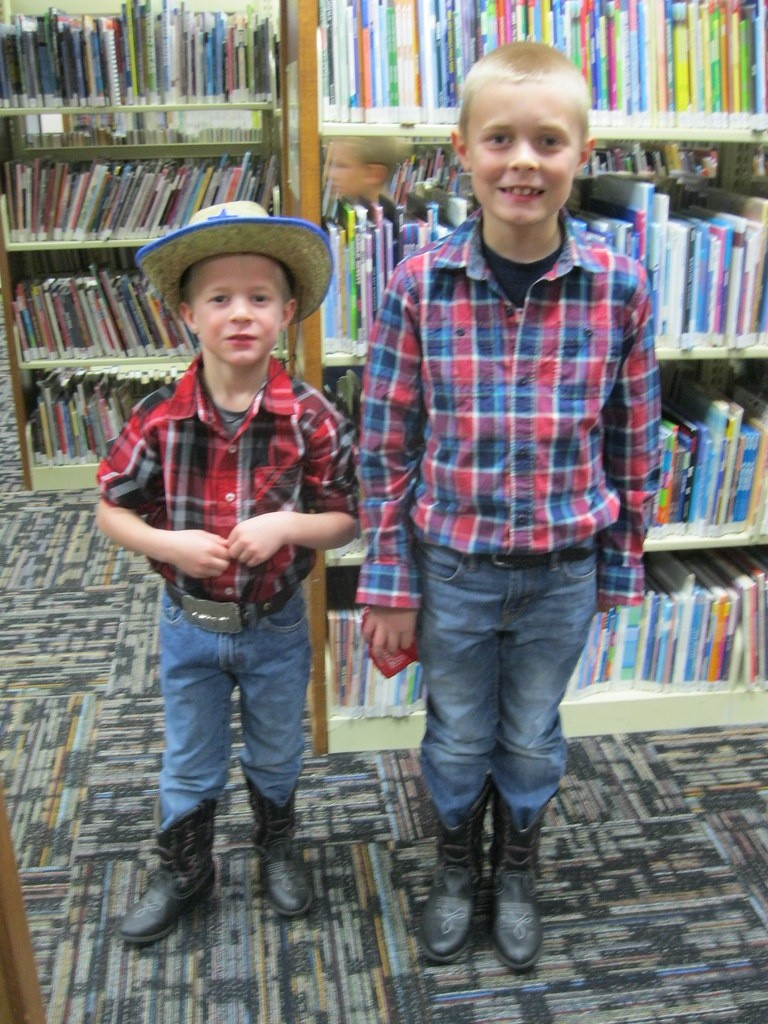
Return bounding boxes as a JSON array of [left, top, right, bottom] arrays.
[[0, 0, 768, 756]]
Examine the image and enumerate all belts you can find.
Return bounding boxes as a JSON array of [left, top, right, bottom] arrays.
[[482, 546, 595, 569], [165, 583, 300, 634]]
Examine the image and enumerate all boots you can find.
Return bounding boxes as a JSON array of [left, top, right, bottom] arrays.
[[420, 774, 494, 961], [116, 796, 218, 941], [245, 773, 312, 917], [488, 783, 561, 971]]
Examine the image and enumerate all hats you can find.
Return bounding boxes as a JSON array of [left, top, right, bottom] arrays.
[[134, 201, 334, 326]]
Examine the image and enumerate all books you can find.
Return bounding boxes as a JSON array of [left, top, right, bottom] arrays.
[[0, 1, 768, 714]]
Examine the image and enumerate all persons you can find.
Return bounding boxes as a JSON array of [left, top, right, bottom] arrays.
[[355, 44, 659, 968], [329, 137, 401, 225], [94, 200, 361, 941]]
